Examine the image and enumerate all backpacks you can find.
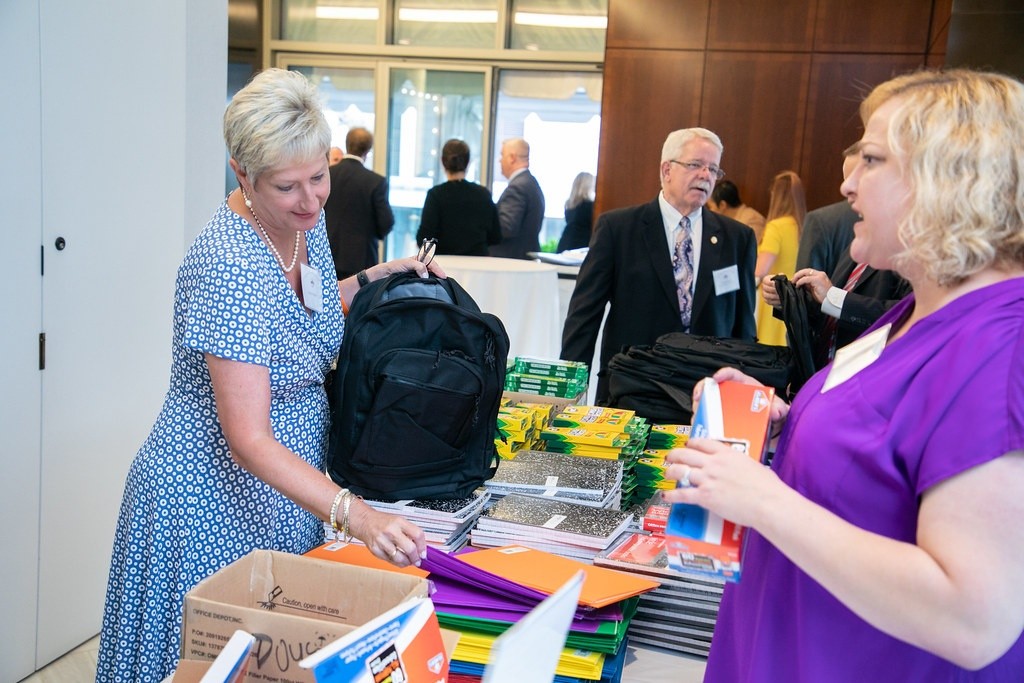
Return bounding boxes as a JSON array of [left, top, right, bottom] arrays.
[[325, 268, 510, 500], [770, 274, 819, 403], [596, 333, 792, 427]]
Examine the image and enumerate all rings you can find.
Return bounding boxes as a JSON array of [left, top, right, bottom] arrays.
[[388, 550, 397, 561], [809, 269, 814, 275], [679, 466, 693, 489]]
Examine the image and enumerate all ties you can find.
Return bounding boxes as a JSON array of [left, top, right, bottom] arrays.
[[672, 216, 694, 333], [829, 264, 869, 363]]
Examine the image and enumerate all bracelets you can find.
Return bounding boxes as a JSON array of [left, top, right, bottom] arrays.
[[329, 486, 349, 541], [339, 495, 365, 543], [357, 269, 371, 288], [343, 491, 351, 542]]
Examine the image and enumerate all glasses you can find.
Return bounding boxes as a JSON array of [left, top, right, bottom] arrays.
[[670, 159, 726, 181], [417, 238, 438, 267]]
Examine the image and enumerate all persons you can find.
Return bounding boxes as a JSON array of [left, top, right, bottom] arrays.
[[556, 172, 596, 278], [708, 142, 912, 376], [658, 67, 1024, 683], [93, 64, 447, 683], [560, 127, 761, 410], [416, 139, 503, 256], [485, 138, 545, 260]]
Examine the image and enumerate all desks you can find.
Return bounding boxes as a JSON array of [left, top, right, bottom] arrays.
[[619, 641, 709, 683], [431, 255, 562, 360]]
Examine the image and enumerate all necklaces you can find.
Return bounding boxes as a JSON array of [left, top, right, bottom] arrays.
[[242, 186, 300, 273]]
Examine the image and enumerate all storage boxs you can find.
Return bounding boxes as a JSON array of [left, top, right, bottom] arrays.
[[158, 547, 460, 683], [664, 377, 776, 585], [502, 355, 693, 511], [644, 505, 671, 532]]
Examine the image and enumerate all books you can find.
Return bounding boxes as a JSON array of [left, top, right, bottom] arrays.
[[481, 571, 586, 683], [526, 252, 583, 266], [199, 628, 256, 683], [299, 594, 449, 683], [300, 377, 776, 682]]
